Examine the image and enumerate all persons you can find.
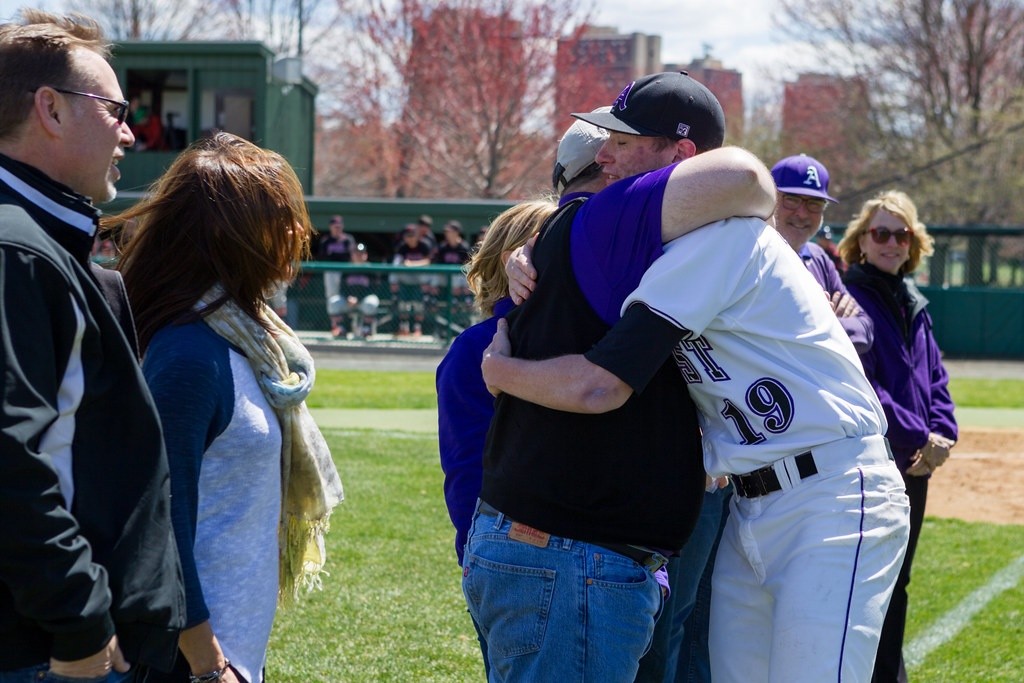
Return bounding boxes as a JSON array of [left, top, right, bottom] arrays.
[[127, 93, 169, 153], [324, 212, 491, 338], [434, 201, 561, 683], [815, 224, 847, 272], [0, 8, 186, 683], [97, 130, 317, 683], [461, 103, 777, 683], [475, 68, 911, 683], [100, 239, 116, 257], [772, 153, 875, 355], [642, 473, 733, 683], [836, 189, 958, 683]]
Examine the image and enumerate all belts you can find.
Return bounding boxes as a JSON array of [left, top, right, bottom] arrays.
[[731, 437, 894, 499], [477, 500, 664, 573]]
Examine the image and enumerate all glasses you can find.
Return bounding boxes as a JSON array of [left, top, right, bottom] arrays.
[[861, 226, 915, 246], [26, 84, 129, 125], [777, 190, 828, 213]]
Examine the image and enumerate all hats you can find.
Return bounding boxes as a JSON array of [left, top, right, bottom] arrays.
[[770, 153, 840, 204], [555, 105, 614, 197], [568, 70, 725, 153]]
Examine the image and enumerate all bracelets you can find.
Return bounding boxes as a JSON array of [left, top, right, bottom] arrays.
[[189, 657, 230, 683]]
